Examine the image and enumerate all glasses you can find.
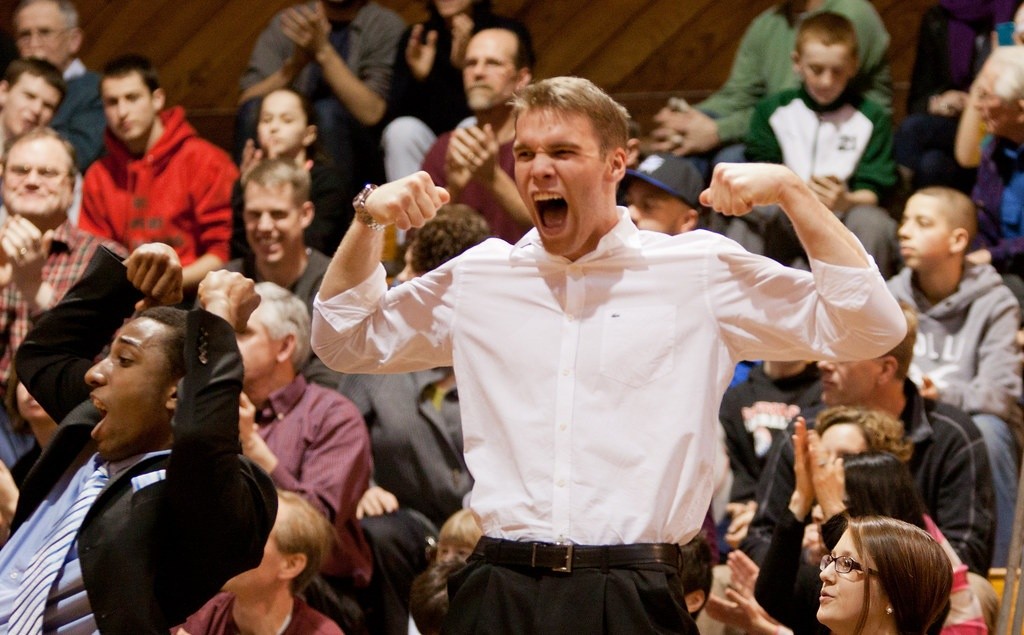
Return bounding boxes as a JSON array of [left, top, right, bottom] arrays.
[[15, 24, 77, 44], [819, 555, 881, 580]]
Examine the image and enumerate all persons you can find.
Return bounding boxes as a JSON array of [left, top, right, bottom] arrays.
[[622, 0, 1024, 635], [0, 126, 129, 400], [14, 0, 107, 176], [0, 55, 66, 182], [244, 0, 403, 148], [381, 0, 497, 183], [396, 204, 488, 285], [229, 88, 354, 262], [0, 242, 343, 635], [338, 366, 483, 635], [308, 77, 908, 635], [235, 281, 372, 635], [191, 157, 342, 390], [420, 16, 536, 246], [79, 54, 240, 288]]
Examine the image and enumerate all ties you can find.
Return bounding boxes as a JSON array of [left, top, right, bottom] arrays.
[[8, 459, 111, 634]]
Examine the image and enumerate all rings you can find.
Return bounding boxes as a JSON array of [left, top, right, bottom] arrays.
[[20, 249, 28, 256]]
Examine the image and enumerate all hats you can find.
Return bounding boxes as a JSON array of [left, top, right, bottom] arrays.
[[622, 154, 705, 208]]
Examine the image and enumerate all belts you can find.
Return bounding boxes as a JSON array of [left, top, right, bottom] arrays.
[[471, 535, 682, 573]]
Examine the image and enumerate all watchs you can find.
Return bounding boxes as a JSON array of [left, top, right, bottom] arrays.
[[352, 184, 387, 231]]
